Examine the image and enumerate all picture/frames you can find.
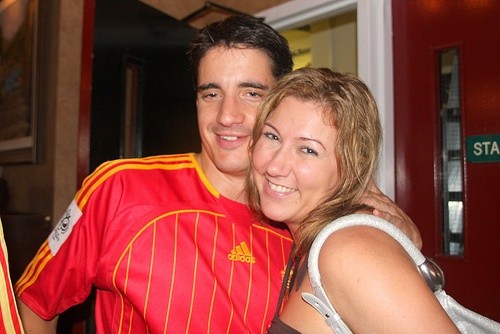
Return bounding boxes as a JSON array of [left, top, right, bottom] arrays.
[[0, 0, 45, 166]]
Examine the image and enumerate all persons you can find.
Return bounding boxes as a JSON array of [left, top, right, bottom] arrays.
[[234, 66, 463, 334], [13, 17, 422, 334]]
